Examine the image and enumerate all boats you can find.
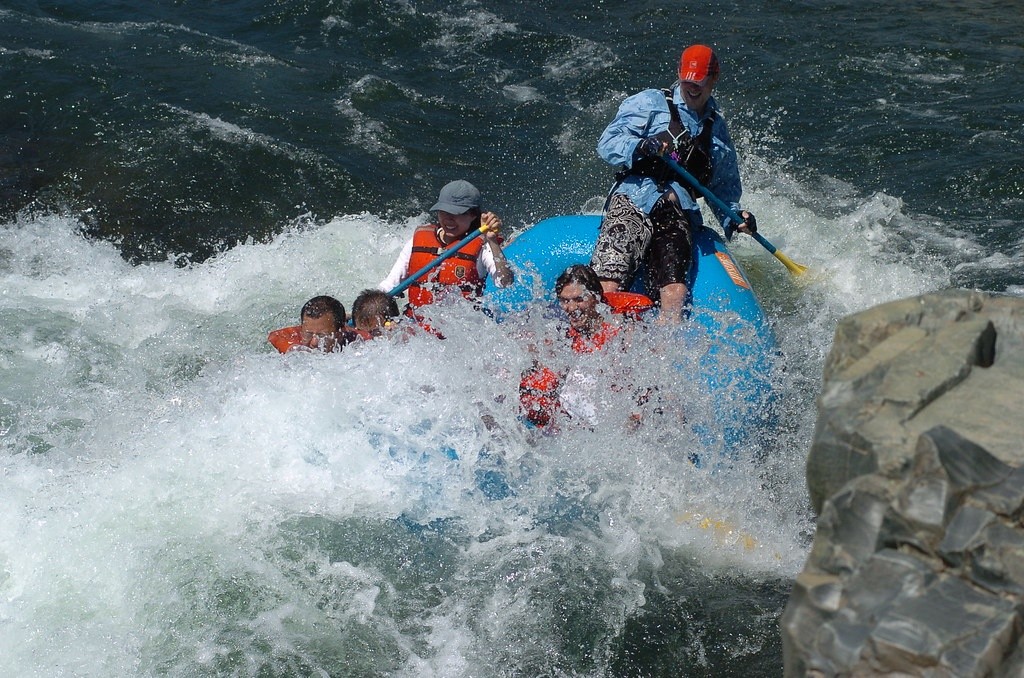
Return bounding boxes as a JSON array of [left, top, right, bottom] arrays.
[[400, 212, 784, 547]]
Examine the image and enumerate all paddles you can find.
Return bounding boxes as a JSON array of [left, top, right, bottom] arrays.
[[341, 215, 493, 330], [644, 137, 811, 287]]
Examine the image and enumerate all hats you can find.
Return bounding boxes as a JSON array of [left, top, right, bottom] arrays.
[[676, 45, 721, 85], [430, 179, 482, 215]]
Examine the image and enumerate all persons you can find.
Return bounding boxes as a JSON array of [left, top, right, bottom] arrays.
[[589, 44, 758, 324], [300, 179, 627, 434]]
